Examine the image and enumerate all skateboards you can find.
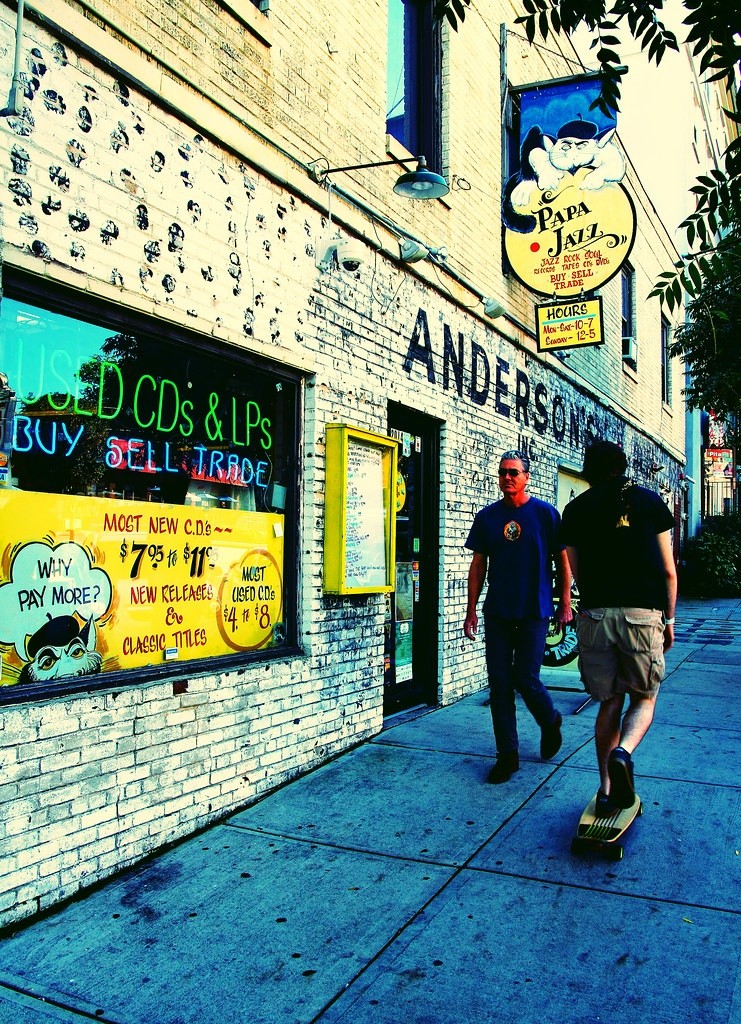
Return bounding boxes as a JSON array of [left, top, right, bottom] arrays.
[[575, 794, 644, 860]]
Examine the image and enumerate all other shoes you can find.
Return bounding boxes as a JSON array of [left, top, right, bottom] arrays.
[[607, 747, 635, 809], [540, 712, 563, 759], [595, 787, 613, 819], [487, 751, 519, 783]]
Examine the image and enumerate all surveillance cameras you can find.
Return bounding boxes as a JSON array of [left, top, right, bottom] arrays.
[[335, 239, 368, 273]]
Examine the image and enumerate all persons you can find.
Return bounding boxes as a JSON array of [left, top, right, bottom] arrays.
[[561, 441, 679, 820], [463, 448, 573, 783]]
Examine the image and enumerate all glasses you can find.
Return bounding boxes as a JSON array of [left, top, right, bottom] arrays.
[[497, 468, 524, 477]]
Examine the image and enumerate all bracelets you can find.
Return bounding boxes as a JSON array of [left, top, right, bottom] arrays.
[[663, 617, 675, 625]]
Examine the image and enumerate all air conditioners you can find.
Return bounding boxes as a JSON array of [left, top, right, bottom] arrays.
[[622, 337, 637, 363]]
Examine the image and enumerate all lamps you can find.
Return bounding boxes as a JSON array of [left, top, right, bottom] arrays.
[[483, 297, 506, 318], [400, 237, 428, 264], [306, 155, 450, 199]]
